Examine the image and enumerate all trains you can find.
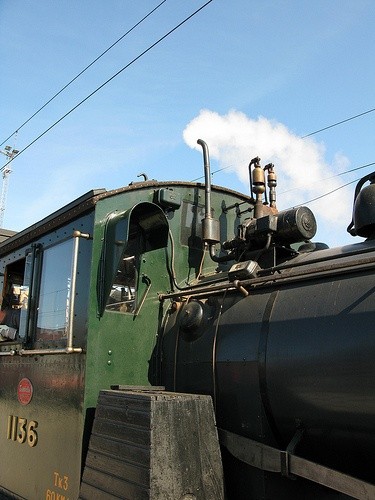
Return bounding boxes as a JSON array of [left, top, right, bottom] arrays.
[[0, 137, 375, 500]]
